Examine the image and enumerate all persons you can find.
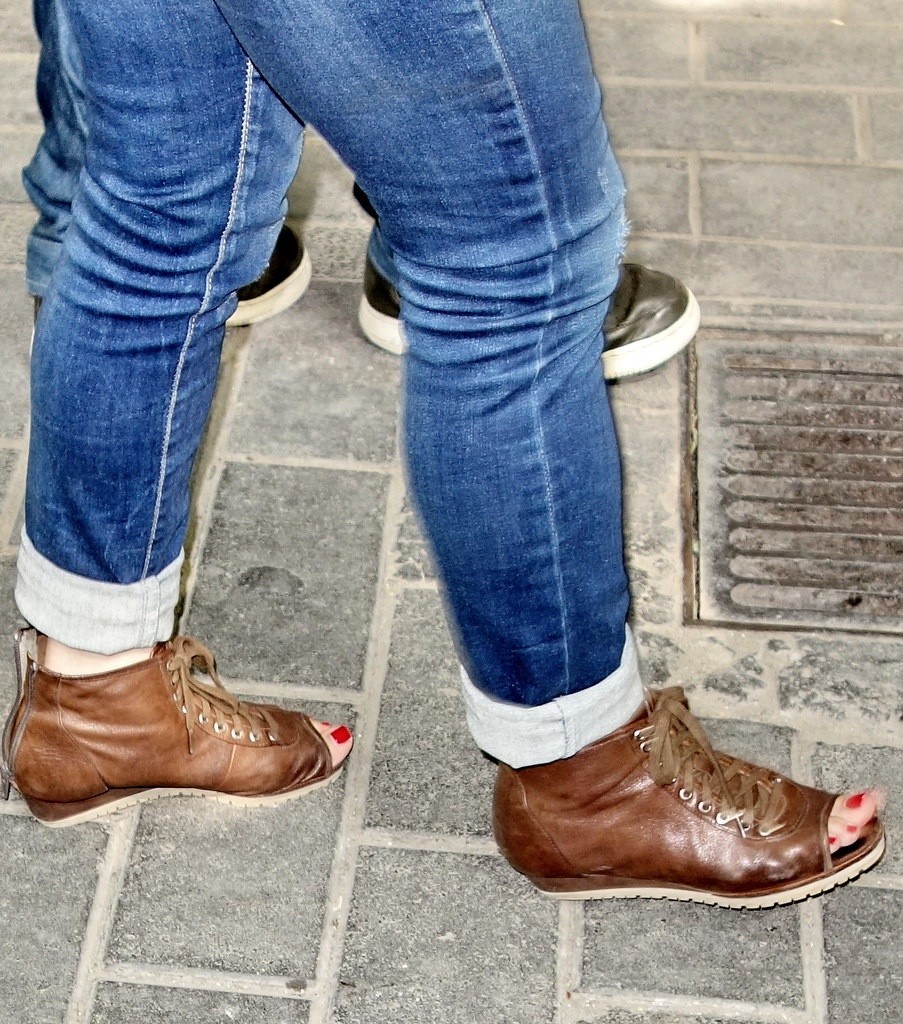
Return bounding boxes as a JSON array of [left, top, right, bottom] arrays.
[[20, 0, 701, 380], [0, 0, 887, 909]]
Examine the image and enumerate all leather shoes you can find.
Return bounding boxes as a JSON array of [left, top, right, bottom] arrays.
[[358, 254, 701, 379], [491, 684, 888, 912], [6, 627, 354, 827], [31, 221, 316, 325]]
